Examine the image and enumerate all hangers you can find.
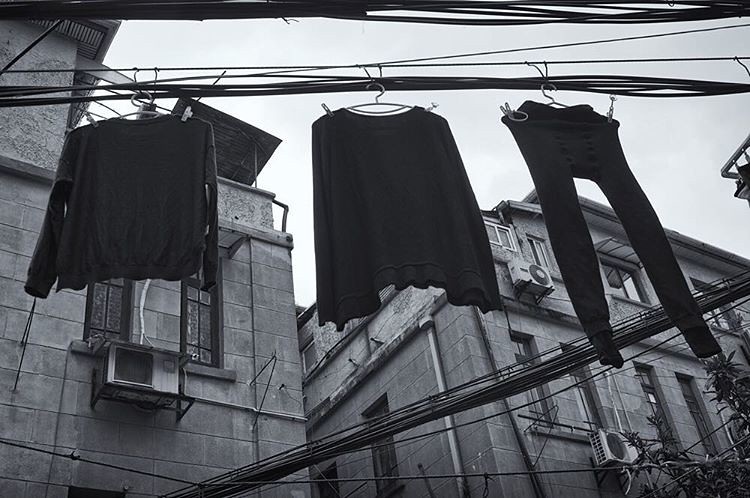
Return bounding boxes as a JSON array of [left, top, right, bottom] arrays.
[[541, 83, 568, 107], [347, 83, 413, 114], [119, 91, 159, 118]]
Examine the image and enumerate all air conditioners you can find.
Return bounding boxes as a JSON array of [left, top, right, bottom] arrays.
[[507, 258, 555, 296], [97, 343, 179, 407], [588, 428, 638, 465]]
